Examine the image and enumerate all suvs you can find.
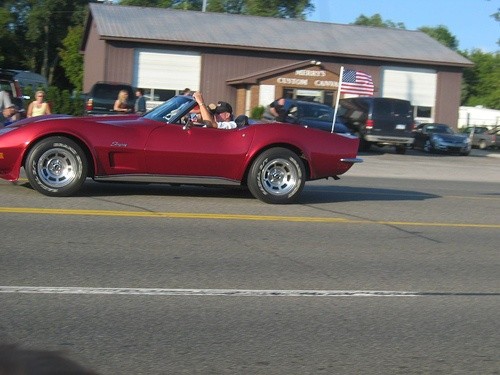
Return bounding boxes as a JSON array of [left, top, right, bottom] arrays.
[[334, 97, 418, 155]]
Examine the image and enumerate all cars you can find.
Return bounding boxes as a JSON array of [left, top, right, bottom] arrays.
[[463, 127, 500, 150], [261, 98, 354, 136], [85, 80, 135, 114], [416, 123, 471, 156], [0, 68, 49, 102]]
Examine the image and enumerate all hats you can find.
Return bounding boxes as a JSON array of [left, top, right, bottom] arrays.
[[210, 101, 232, 113]]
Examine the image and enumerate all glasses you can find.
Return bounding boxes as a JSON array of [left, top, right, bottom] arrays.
[[212, 112, 222, 114]]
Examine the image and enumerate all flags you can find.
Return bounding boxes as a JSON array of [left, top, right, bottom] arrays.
[[340, 69, 375, 96]]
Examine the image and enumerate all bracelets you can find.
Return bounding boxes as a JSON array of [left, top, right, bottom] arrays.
[[199, 102, 205, 105]]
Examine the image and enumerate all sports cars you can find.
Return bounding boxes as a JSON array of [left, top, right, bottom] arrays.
[[0, 95, 360, 205]]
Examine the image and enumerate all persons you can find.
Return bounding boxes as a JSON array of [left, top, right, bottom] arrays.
[[0, 90, 25, 117], [26, 90, 52, 119], [113, 89, 133, 113], [134, 87, 147, 114], [192, 91, 238, 130], [180, 88, 218, 119]]
